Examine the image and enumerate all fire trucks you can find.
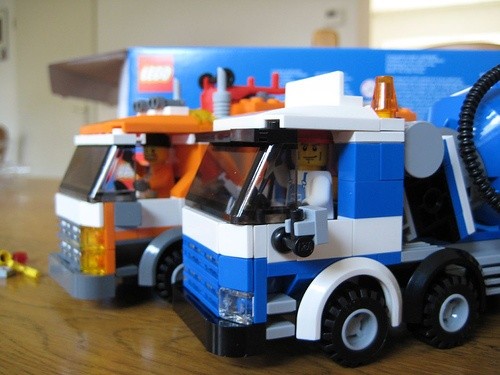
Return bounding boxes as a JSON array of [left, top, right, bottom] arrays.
[[48, 66, 420, 306], [172, 70, 500, 368]]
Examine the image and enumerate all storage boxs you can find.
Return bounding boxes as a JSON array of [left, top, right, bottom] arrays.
[[48, 43, 500, 121]]
